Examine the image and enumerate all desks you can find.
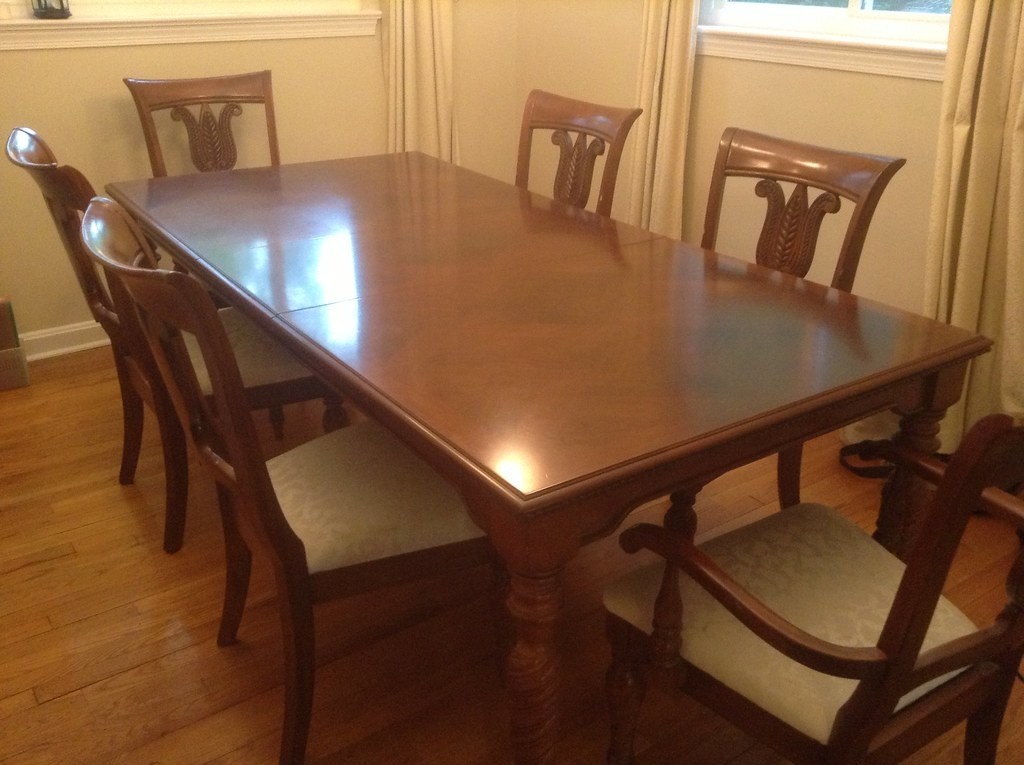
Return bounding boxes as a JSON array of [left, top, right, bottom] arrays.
[[108, 152, 990, 765]]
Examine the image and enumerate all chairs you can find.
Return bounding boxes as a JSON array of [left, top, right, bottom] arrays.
[[83, 195, 503, 765], [511, 89, 645, 216], [125, 70, 281, 306], [597, 418, 1024, 765], [699, 124, 907, 510], [5, 129, 346, 552]]
